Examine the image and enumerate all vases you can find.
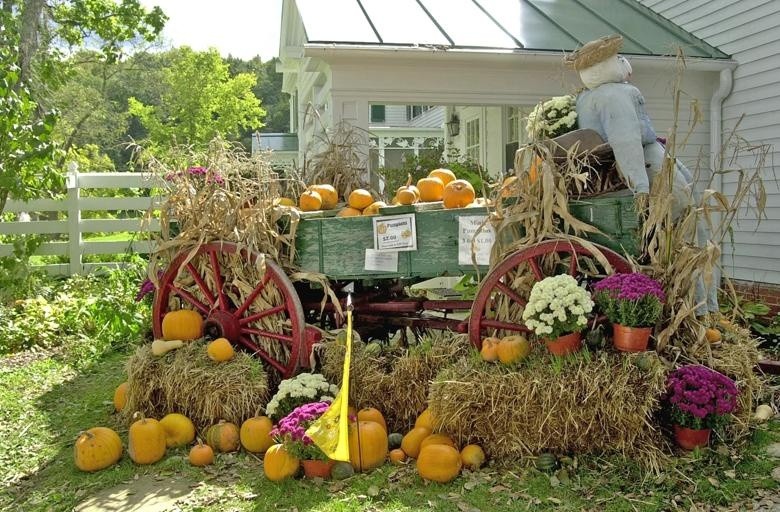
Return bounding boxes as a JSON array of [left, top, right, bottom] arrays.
[[538, 330, 585, 357], [672, 426, 714, 450], [302, 458, 343, 482], [611, 322, 654, 353]]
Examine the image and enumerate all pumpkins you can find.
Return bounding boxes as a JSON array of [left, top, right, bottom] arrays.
[[537, 453, 558, 471], [280, 158, 543, 217], [162, 310, 203, 339], [481, 336, 529, 363], [74, 382, 485, 483], [207, 338, 234, 360], [706, 327, 722, 342], [719, 320, 731, 325]]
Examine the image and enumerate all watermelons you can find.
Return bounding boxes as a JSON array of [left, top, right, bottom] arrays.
[[365, 342, 382, 357]]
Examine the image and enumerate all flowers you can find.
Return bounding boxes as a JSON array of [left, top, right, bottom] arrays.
[[522, 274, 596, 337], [658, 365, 739, 425], [265, 372, 341, 417], [592, 271, 667, 326], [525, 96, 581, 141], [266, 401, 367, 461]]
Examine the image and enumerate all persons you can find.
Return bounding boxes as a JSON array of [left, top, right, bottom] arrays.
[[561, 33, 723, 341]]
